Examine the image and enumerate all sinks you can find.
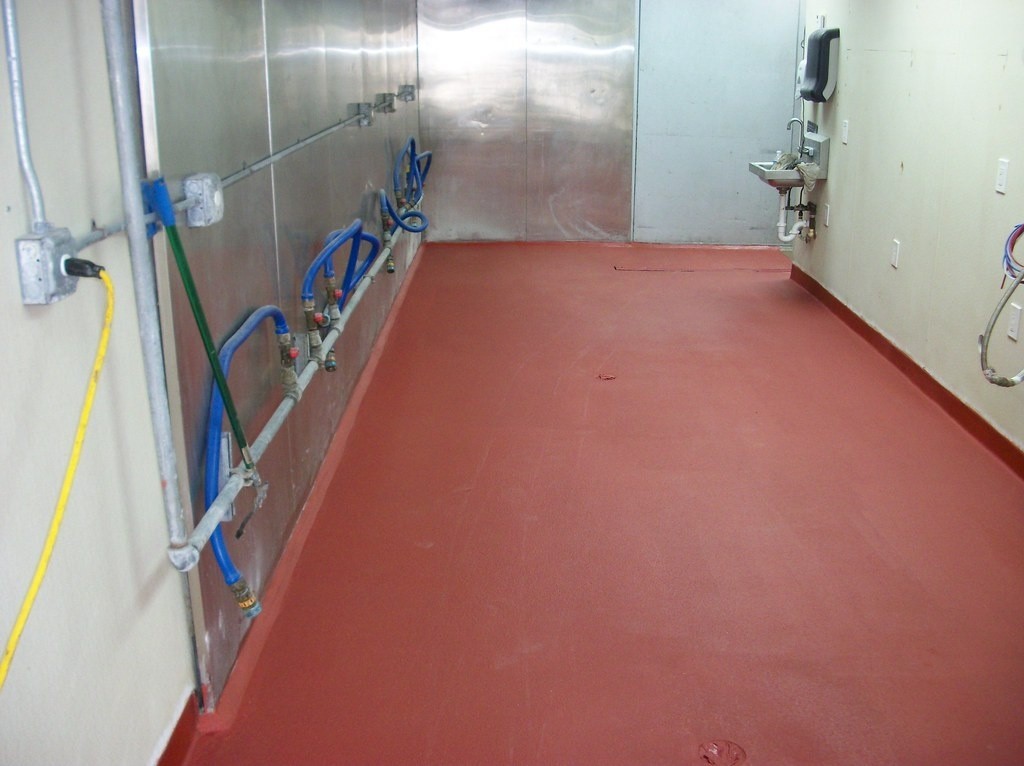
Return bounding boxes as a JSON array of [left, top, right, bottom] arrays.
[[748, 162, 828, 189]]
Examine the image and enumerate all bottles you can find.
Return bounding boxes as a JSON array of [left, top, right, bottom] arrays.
[[773, 150, 781, 163]]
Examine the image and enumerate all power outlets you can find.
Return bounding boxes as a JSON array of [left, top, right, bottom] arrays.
[[16, 227, 83, 306], [185, 172, 225, 227]]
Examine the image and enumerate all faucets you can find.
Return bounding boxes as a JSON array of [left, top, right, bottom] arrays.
[[787, 118, 809, 158]]
